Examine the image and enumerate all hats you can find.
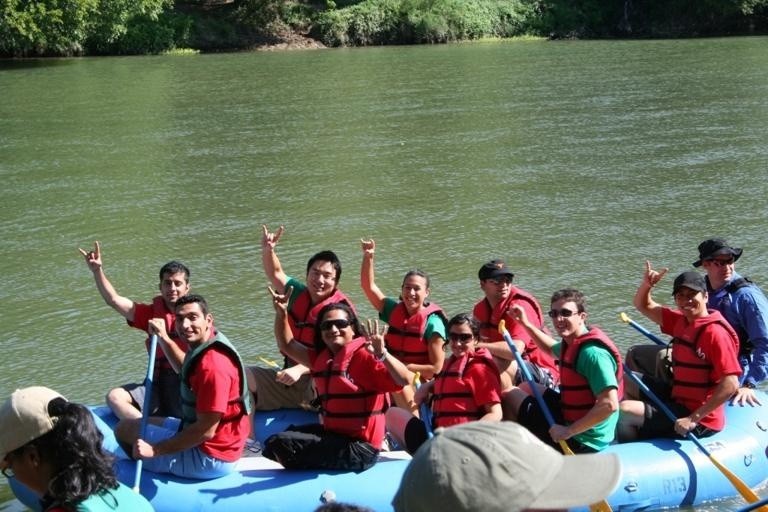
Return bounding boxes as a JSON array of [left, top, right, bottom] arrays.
[[692, 238, 743, 266], [0, 385, 69, 463], [672, 272, 707, 295], [478, 259, 514, 281]]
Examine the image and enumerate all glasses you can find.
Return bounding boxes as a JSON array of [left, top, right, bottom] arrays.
[[3, 446, 34, 477], [447, 332, 473, 344], [708, 257, 736, 265], [548, 308, 579, 317], [319, 320, 353, 331]]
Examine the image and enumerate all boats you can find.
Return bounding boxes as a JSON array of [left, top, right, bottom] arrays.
[[82, 384, 768, 509]]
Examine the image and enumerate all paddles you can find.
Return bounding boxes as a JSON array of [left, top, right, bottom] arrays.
[[622, 361, 768, 512], [499, 319, 611, 512]]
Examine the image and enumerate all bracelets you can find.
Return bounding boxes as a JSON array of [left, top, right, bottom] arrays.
[[373, 348, 387, 363]]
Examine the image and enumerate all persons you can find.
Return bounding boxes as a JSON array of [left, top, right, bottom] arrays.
[[633, 260, 742, 440], [115, 295, 251, 481], [244, 224, 358, 412], [501, 287, 625, 457], [359, 235, 447, 378], [0, 386, 154, 512], [314, 501, 374, 512], [473, 259, 560, 389], [384, 312, 503, 454], [79, 240, 191, 419], [691, 238, 768, 409], [263, 284, 411, 472]]
